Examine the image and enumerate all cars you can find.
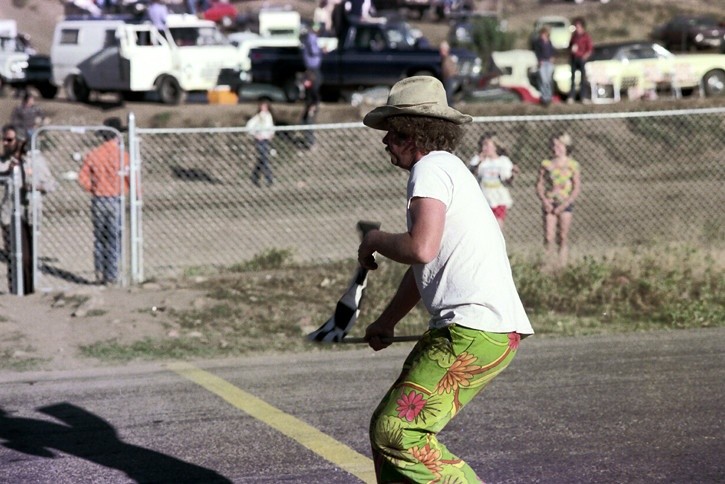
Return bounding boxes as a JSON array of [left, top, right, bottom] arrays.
[[650, 15, 724, 51], [528, 15, 575, 50], [449, 12, 499, 48], [552, 40, 725, 99]]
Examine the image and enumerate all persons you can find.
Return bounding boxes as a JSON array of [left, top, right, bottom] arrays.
[[537, 131, 582, 266], [63, 0, 238, 30], [0, 91, 58, 291], [466, 131, 519, 231], [362, 75, 534, 484], [534, 17, 594, 108], [247, 102, 273, 187], [78, 116, 131, 285], [299, 1, 480, 149]]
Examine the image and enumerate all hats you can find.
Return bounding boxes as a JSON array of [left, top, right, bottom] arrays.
[[363, 76, 474, 131], [95, 118, 128, 136]]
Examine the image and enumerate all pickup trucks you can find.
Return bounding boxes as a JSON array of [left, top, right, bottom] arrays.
[[248, 23, 484, 102]]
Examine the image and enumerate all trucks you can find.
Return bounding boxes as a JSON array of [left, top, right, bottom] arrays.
[[51, 13, 252, 106], [0, 20, 61, 100]]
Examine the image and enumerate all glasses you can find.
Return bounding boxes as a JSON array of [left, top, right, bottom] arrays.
[[3, 138, 15, 142]]
